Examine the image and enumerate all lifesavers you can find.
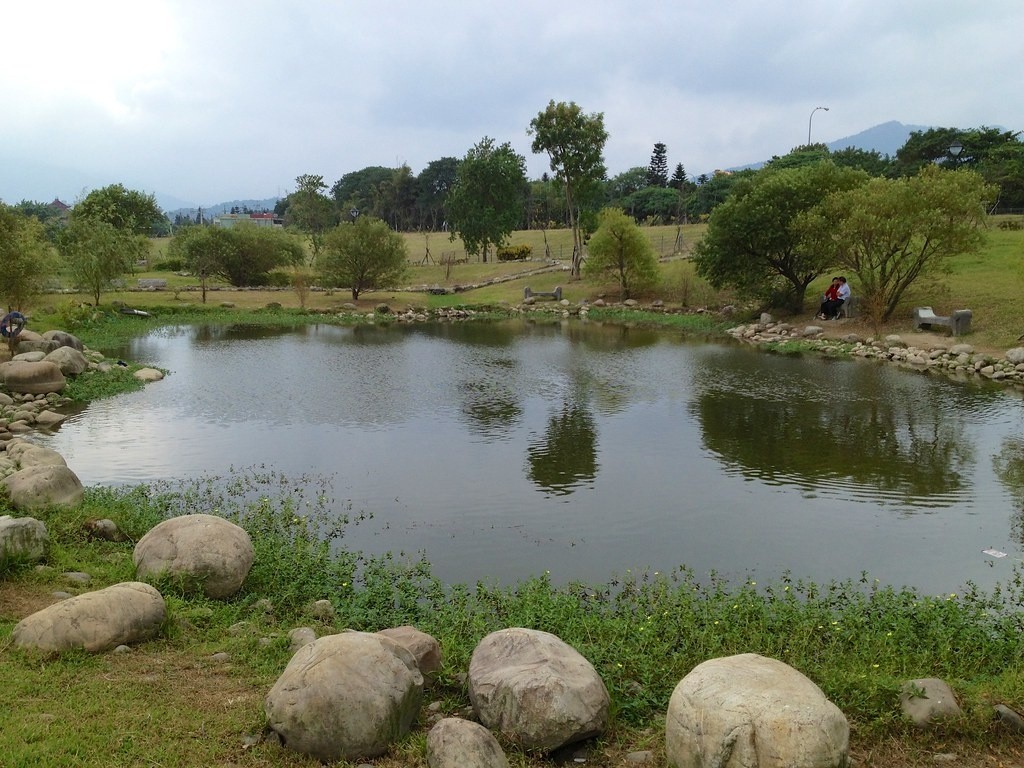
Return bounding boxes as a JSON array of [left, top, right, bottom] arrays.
[[0, 311, 25, 338]]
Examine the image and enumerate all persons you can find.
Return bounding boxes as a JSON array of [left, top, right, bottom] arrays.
[[816, 276, 850, 321]]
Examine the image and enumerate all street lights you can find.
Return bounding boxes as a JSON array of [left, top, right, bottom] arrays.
[[808, 106, 829, 146]]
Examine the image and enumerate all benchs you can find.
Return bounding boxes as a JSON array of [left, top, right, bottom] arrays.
[[819, 296, 853, 317], [523, 286, 563, 301], [913, 306, 972, 336]]
